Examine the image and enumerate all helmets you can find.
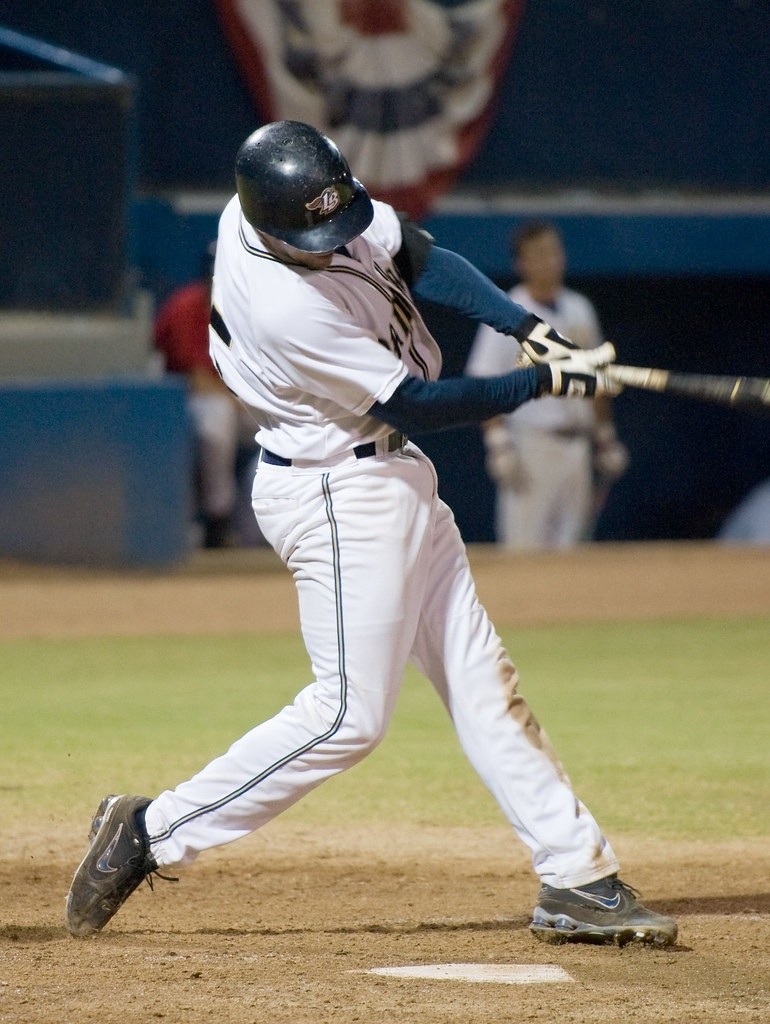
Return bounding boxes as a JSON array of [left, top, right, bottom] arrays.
[[236, 120, 374, 254]]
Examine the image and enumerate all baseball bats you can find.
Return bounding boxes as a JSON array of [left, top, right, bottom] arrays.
[[517, 352, 770, 408]]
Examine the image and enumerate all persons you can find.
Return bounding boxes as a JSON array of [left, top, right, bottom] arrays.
[[155, 277, 238, 521], [66, 121, 677, 944], [466, 228, 633, 544]]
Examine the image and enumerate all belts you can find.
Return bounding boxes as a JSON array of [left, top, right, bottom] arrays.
[[262, 429, 408, 466]]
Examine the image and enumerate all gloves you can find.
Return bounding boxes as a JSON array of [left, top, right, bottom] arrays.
[[514, 314, 623, 401], [486, 434, 522, 487], [596, 429, 628, 479]]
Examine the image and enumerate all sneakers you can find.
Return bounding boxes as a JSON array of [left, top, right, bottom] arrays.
[[64, 794, 179, 938], [530, 871, 678, 948]]
